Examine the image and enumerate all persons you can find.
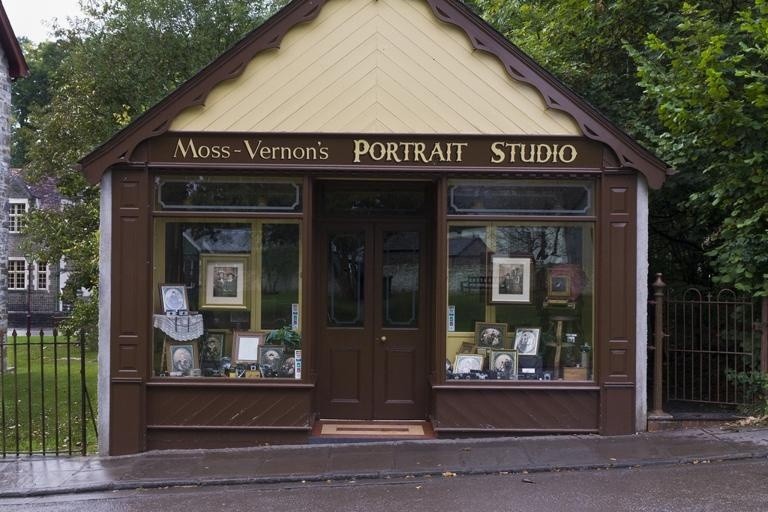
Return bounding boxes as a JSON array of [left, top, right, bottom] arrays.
[[503, 266, 523, 294], [215, 270, 236, 297]]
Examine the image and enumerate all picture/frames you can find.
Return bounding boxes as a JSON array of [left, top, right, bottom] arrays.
[[487, 251, 536, 306], [159, 282, 188, 315], [165, 329, 287, 376], [453, 321, 541, 379], [546, 268, 572, 298], [198, 253, 253, 312]]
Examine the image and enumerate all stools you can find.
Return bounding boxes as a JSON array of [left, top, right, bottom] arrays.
[[542, 314, 588, 380]]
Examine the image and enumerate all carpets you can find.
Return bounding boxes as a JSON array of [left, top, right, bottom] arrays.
[[312, 419, 438, 440]]
